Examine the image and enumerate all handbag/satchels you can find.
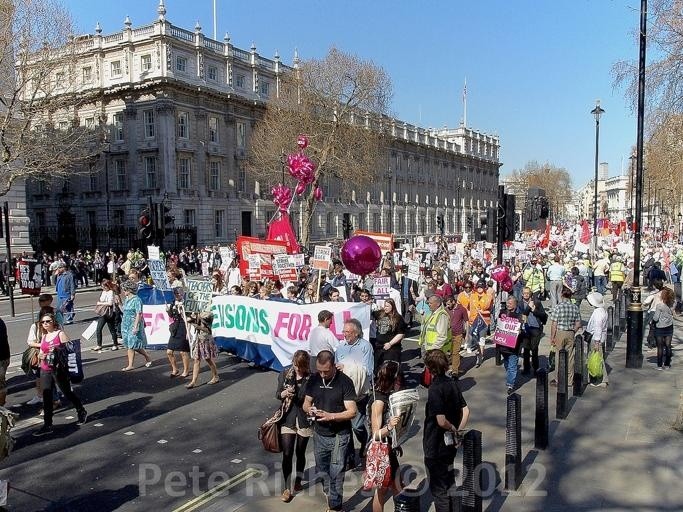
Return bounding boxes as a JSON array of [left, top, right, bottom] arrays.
[[364, 441, 391, 492], [588, 349, 603, 377], [258, 413, 281, 453], [646, 321, 656, 348], [21, 348, 41, 377], [96, 305, 108, 316], [547, 346, 554, 372], [582, 330, 592, 343]]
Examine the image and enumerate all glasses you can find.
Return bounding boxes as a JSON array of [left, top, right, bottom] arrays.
[[41, 320, 51, 323]]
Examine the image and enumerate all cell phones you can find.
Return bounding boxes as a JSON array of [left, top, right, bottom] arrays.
[[307, 410, 318, 416]]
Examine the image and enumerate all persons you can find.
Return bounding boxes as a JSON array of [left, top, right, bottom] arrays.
[[0, 222, 683, 512]]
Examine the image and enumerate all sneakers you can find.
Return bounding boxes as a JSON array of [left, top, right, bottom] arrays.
[[207, 376, 219, 384], [94, 346, 101, 351], [121, 367, 133, 371], [185, 383, 193, 389], [281, 489, 290, 501], [76, 408, 88, 426], [146, 355, 152, 368], [171, 369, 188, 378], [27, 396, 43, 405], [112, 346, 117, 350], [33, 426, 53, 436]]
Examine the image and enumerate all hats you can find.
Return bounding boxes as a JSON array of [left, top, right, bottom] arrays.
[[475, 281, 484, 291], [586, 292, 604, 308]]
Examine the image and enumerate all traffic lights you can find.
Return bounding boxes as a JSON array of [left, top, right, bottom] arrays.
[[138, 209, 152, 241], [155, 203, 181, 237], [342, 218, 348, 232], [348, 219, 353, 230], [436, 214, 441, 228], [477, 207, 495, 244], [467, 215, 472, 228], [506, 194, 516, 239]]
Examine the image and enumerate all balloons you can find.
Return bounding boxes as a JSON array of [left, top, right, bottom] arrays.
[[271, 133, 323, 212]]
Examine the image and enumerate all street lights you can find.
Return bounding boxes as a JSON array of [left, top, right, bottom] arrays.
[[629, 154, 669, 237], [385, 166, 392, 235], [455, 181, 462, 234], [583, 99, 606, 251], [100, 137, 112, 248], [278, 147, 287, 186]]
